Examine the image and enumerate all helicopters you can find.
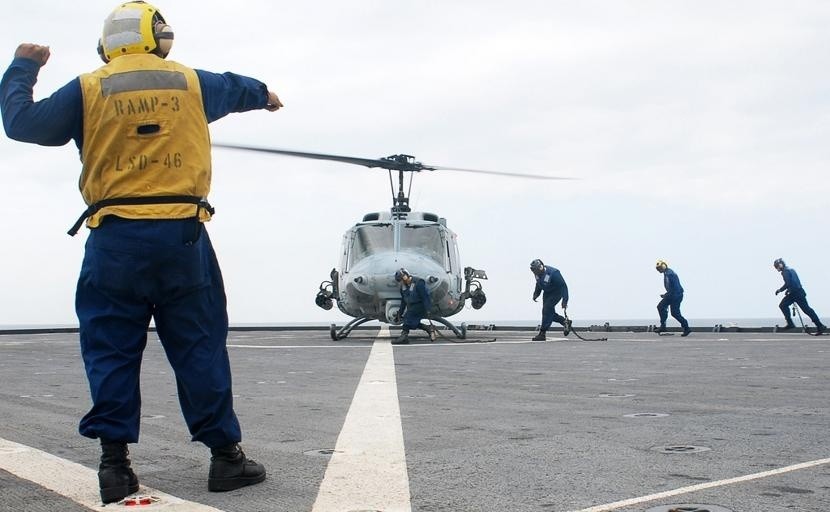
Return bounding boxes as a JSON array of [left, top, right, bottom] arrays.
[[209, 144, 573, 341]]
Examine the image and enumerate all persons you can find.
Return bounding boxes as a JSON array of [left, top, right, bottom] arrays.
[[530, 259, 572, 341], [0, 0, 284, 504], [654, 259, 692, 336], [390, 267, 436, 345], [774, 258, 827, 336]]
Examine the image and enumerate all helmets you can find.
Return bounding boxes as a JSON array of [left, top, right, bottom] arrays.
[[774, 258, 785, 272], [101, 0, 165, 61], [656, 260, 667, 273], [530, 259, 545, 276]]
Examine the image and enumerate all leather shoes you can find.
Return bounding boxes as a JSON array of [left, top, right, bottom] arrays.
[[97, 459, 139, 503], [563, 320, 572, 336], [207, 452, 266, 491], [531, 333, 546, 341], [681, 327, 692, 336], [653, 326, 667, 332]]
